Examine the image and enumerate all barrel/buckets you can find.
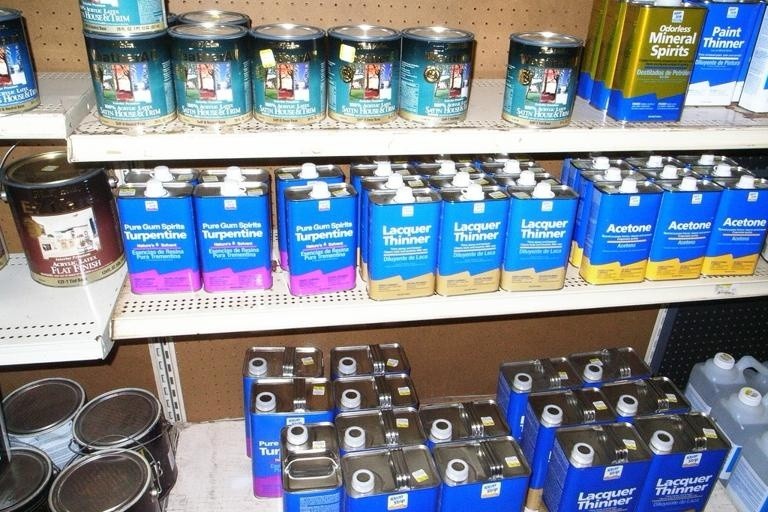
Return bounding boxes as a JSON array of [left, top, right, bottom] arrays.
[[243, 344, 532, 512], [399, 25, 475, 122], [0, 381, 180, 512], [0, 9, 41, 114], [115, 167, 275, 294], [579, 0, 768, 127], [273, 155, 580, 302], [79, 2, 252, 128], [325, 24, 401, 126], [498, 346, 734, 512], [1, 231, 11, 270], [253, 22, 329, 122], [0, 150, 126, 289], [684, 351, 768, 512], [560, 153, 767, 286], [500, 30, 584, 130]]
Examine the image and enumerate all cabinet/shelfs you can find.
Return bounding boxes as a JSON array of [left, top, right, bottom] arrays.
[[1, 66, 768, 427]]
